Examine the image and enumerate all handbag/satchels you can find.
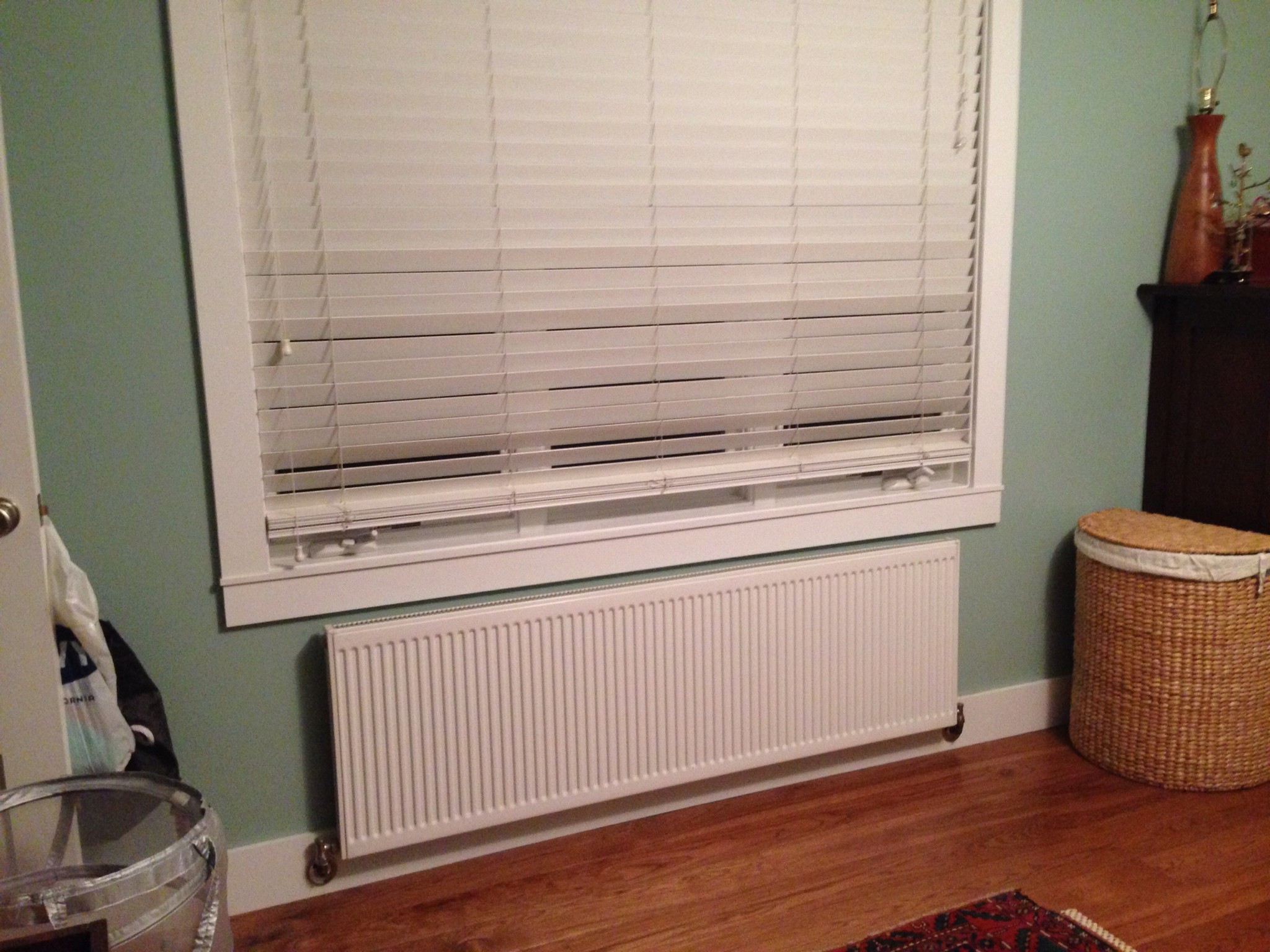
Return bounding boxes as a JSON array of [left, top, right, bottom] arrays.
[[74, 621, 182, 847], [42, 516, 137, 779]]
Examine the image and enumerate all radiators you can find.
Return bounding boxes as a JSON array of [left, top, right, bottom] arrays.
[[304, 538, 966, 887]]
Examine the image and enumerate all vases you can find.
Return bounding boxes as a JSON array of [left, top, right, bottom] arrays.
[[1162, 113, 1229, 283]]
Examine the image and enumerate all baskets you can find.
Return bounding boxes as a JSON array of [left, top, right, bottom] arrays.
[[1068, 506, 1270, 791]]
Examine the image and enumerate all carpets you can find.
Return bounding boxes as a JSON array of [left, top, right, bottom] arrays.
[[821, 890, 1141, 952]]
